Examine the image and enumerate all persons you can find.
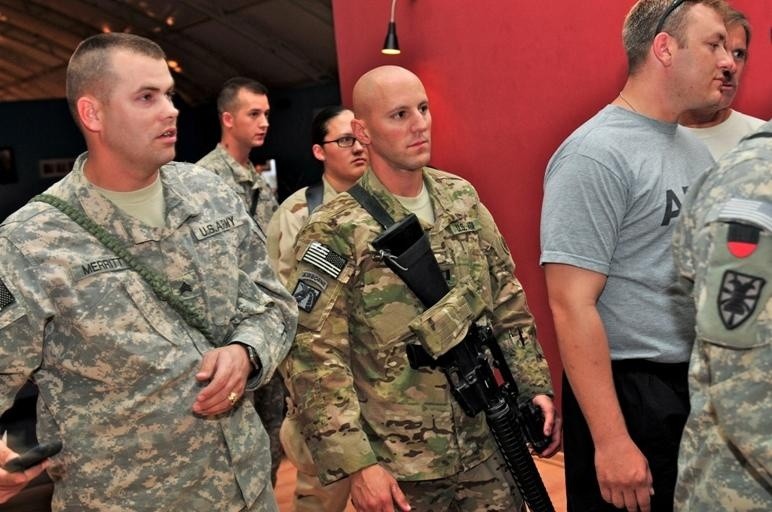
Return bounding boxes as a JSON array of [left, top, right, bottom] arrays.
[[677, 4, 768, 163], [195, 77, 291, 490], [285, 65, 563, 512], [673, 119, 772, 512], [0, 33, 300, 512], [539, 0, 734, 512], [265, 108, 370, 512]]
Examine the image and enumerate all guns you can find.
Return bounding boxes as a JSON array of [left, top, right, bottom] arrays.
[[372, 213, 558, 511]]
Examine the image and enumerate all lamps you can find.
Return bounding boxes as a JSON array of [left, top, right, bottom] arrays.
[[380, 0, 401, 56]]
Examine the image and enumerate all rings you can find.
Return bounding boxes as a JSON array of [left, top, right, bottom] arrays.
[[228, 390, 238, 407]]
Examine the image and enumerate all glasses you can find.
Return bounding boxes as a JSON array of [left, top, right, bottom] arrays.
[[319, 137, 356, 147]]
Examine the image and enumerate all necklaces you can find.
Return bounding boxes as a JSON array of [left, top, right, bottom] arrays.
[[619, 92, 638, 114]]
